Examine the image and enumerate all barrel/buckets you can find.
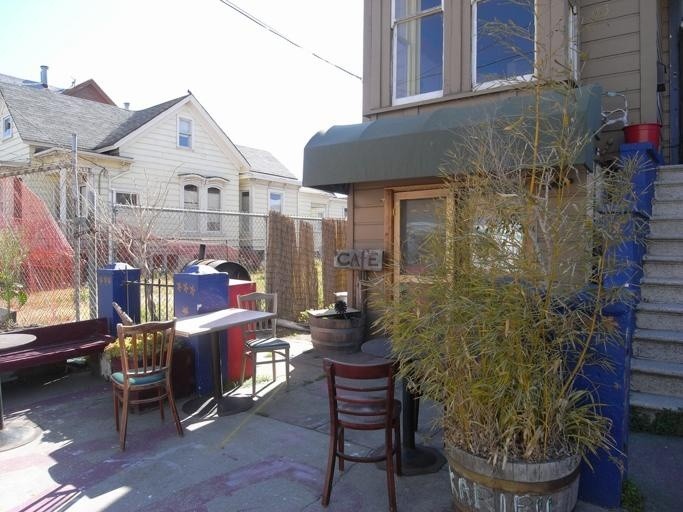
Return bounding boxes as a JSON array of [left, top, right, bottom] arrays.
[[440, 429, 582, 512], [623, 122, 663, 151], [307, 314, 364, 358], [181, 258, 251, 281]]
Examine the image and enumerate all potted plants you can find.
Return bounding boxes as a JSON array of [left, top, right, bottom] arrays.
[[360, 0, 658, 512], [587, 88, 662, 151]]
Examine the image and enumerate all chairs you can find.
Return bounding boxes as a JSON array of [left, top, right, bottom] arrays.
[[110, 292, 290, 452], [320, 355, 401, 511]]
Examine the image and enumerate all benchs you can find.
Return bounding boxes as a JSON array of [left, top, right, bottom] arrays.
[[0, 316, 118, 382]]
